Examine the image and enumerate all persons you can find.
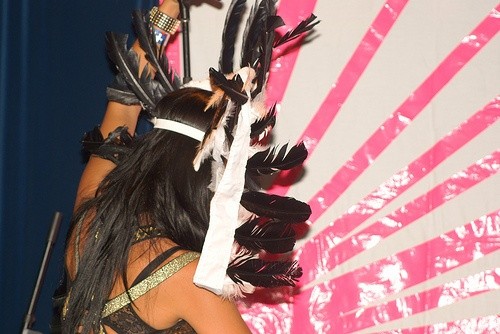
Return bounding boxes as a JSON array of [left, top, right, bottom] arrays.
[[60, 1, 313, 334]]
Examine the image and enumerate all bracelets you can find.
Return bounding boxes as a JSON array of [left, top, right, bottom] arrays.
[[149, 6, 182, 35], [146, 24, 171, 49]]
